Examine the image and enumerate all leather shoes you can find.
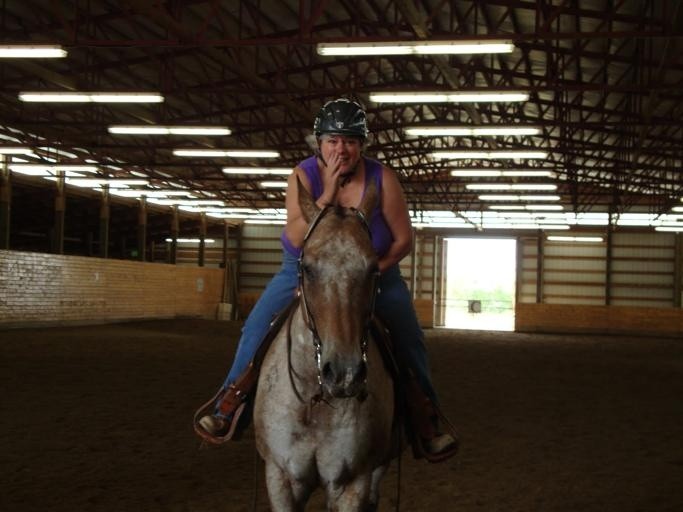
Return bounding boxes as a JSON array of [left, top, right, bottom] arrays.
[[199, 414, 245, 439], [413, 434, 456, 465]]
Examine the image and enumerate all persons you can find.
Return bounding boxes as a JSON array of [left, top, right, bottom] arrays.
[[190, 99, 461, 464]]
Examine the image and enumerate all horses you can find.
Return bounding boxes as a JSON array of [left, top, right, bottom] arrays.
[[253, 172, 395, 512]]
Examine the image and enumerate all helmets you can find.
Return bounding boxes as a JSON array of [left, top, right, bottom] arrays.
[[314, 99, 369, 146]]
